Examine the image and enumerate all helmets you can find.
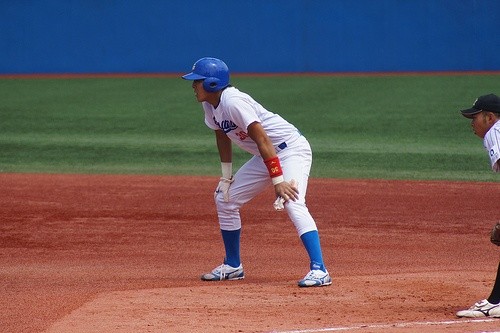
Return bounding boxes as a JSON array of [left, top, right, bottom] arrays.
[[182, 57, 230, 93]]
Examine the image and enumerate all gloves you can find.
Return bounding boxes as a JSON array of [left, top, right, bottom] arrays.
[[214, 177, 234, 203], [273, 180, 296, 211]]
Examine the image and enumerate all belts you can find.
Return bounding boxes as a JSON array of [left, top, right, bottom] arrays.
[[278, 142, 287, 150]]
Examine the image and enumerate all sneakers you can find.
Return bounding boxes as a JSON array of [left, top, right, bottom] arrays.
[[201, 264, 244, 281], [298, 270, 332, 287], [457, 299, 500, 318]]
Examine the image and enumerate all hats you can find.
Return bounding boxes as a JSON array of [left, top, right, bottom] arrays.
[[461, 94, 500, 118]]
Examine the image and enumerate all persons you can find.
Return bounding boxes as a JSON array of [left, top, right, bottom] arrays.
[[181, 56, 331, 287], [456, 92, 500, 319]]
